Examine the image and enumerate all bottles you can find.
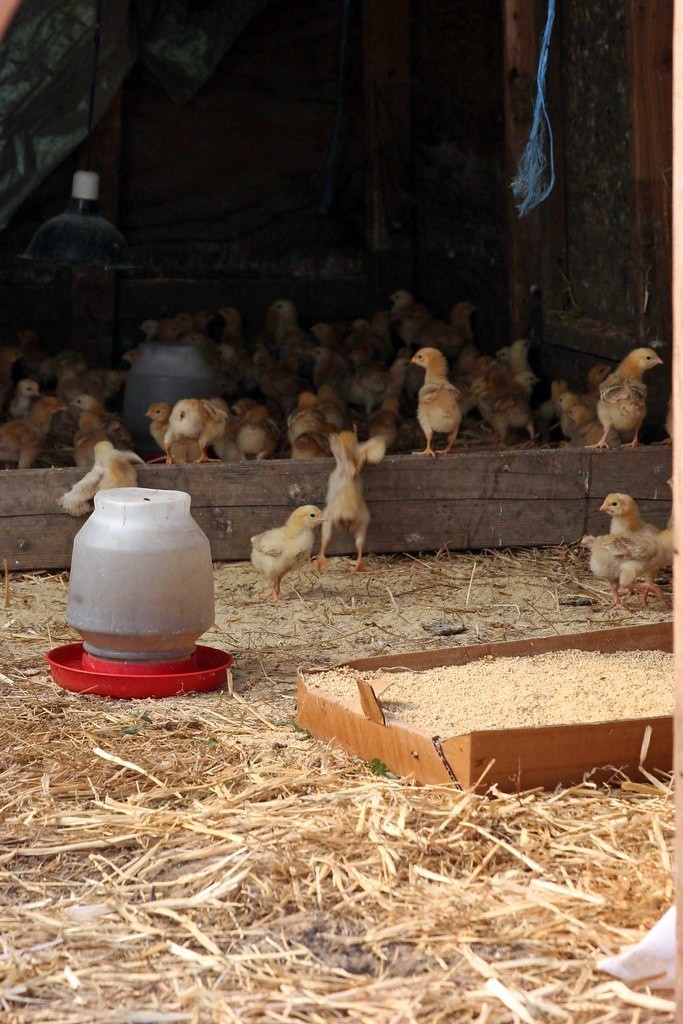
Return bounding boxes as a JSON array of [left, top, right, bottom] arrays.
[[20, 170, 130, 273]]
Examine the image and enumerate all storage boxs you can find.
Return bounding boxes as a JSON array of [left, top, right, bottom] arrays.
[[295, 621, 673, 799], [0, 270, 671, 573]]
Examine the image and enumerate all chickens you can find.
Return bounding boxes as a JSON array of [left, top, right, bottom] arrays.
[[251, 505, 328, 606], [312, 430, 388, 573], [579, 529, 673, 617], [1, 290, 673, 468], [55, 439, 150, 518], [599, 491, 674, 610]]
